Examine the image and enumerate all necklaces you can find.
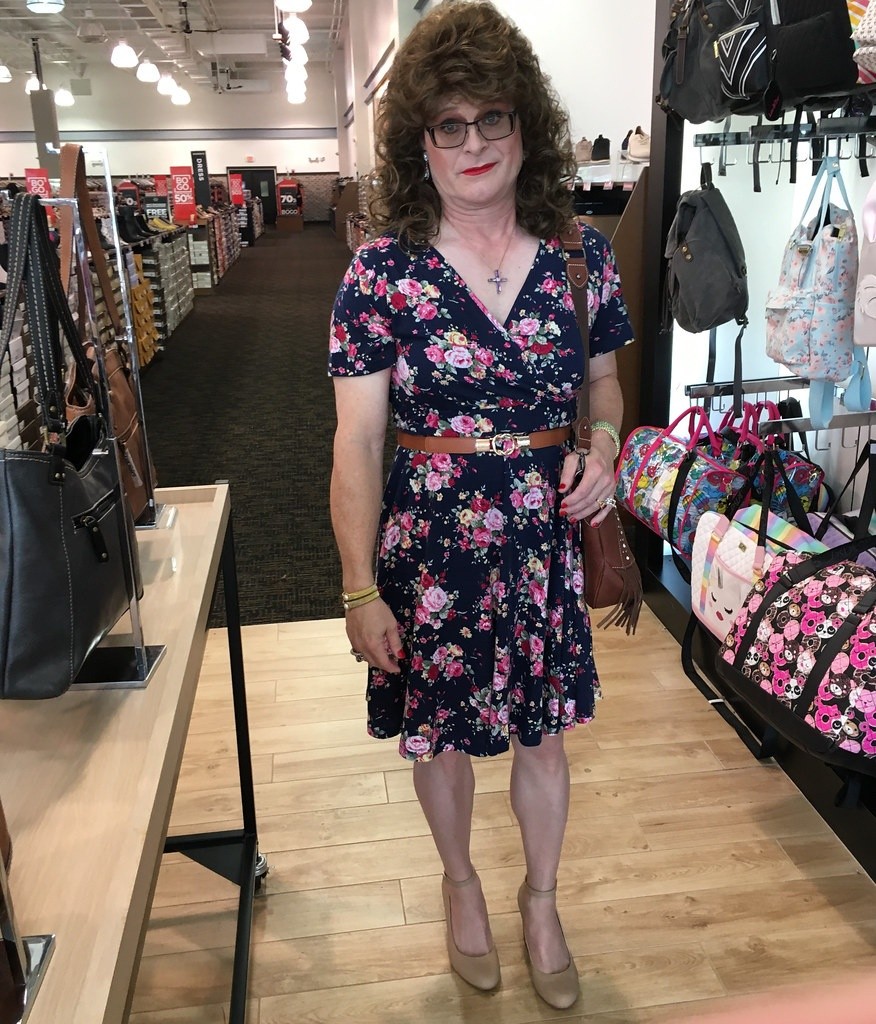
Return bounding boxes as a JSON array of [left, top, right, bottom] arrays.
[[442, 213, 517, 295]]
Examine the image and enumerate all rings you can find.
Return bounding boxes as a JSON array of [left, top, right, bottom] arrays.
[[597, 500, 605, 510], [605, 498, 617, 509], [351, 648, 366, 662]]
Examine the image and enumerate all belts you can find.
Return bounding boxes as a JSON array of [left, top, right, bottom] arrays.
[[398, 426, 571, 455]]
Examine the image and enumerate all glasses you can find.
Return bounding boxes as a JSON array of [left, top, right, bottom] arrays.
[[424, 108, 518, 148]]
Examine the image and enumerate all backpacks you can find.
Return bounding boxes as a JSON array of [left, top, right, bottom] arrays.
[[652, 0, 876, 127], [661, 162, 750, 333]]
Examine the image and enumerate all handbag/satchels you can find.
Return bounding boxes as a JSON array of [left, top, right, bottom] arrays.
[[614, 398, 876, 779], [57, 142, 158, 523], [572, 474, 643, 635], [851, 181, 876, 348], [0, 193, 145, 702], [764, 156, 858, 382]]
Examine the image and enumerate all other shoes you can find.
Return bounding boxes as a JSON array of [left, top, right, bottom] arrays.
[[0, 182, 237, 290], [565, 123, 651, 163]]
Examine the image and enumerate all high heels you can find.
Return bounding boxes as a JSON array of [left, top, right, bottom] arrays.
[[442, 863, 500, 991], [517, 874, 580, 1010]]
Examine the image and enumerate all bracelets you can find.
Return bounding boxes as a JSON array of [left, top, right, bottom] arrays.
[[591, 421, 620, 460], [341, 584, 380, 610]]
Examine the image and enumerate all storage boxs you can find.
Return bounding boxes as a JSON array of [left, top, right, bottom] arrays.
[[344, 182, 379, 254], [0, 0, 313, 108], [1, 191, 261, 453]]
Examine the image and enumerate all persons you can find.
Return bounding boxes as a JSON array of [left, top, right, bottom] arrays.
[[323, 0, 636, 1009]]
